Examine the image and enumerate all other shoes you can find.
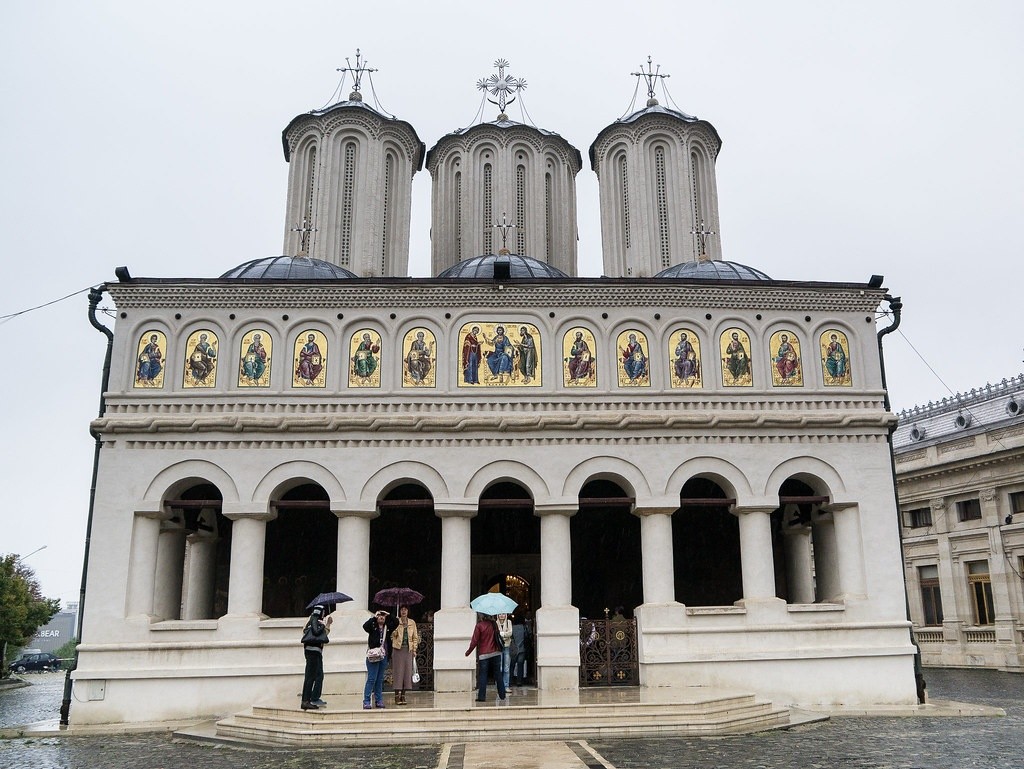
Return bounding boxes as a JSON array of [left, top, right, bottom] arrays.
[[500, 697, 505, 700], [395, 695, 408, 705], [504, 687, 512, 693], [301, 699, 328, 709], [475, 699, 485, 702], [510, 682, 522, 687]]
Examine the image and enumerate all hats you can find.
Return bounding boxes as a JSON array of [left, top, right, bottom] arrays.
[[314, 604, 325, 620]]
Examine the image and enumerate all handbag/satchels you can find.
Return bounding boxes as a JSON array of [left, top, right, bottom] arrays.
[[411, 673, 421, 684], [365, 647, 386, 663], [514, 660, 528, 678], [510, 640, 519, 659], [494, 631, 505, 652]]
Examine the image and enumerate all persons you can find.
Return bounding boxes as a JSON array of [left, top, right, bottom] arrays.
[[363, 612, 399, 708], [390, 606, 417, 704], [610, 605, 627, 647], [465, 612, 506, 702], [301, 605, 333, 708], [579, 610, 595, 682], [497, 611, 535, 693]]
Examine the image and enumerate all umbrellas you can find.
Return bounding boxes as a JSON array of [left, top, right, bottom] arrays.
[[471, 592, 518, 616], [374, 587, 424, 617], [306, 592, 354, 615]]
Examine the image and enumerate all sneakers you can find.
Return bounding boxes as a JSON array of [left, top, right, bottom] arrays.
[[364, 699, 371, 710], [376, 700, 385, 708]]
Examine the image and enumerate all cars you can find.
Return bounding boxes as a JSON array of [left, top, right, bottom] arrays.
[[9, 653, 61, 674]]
